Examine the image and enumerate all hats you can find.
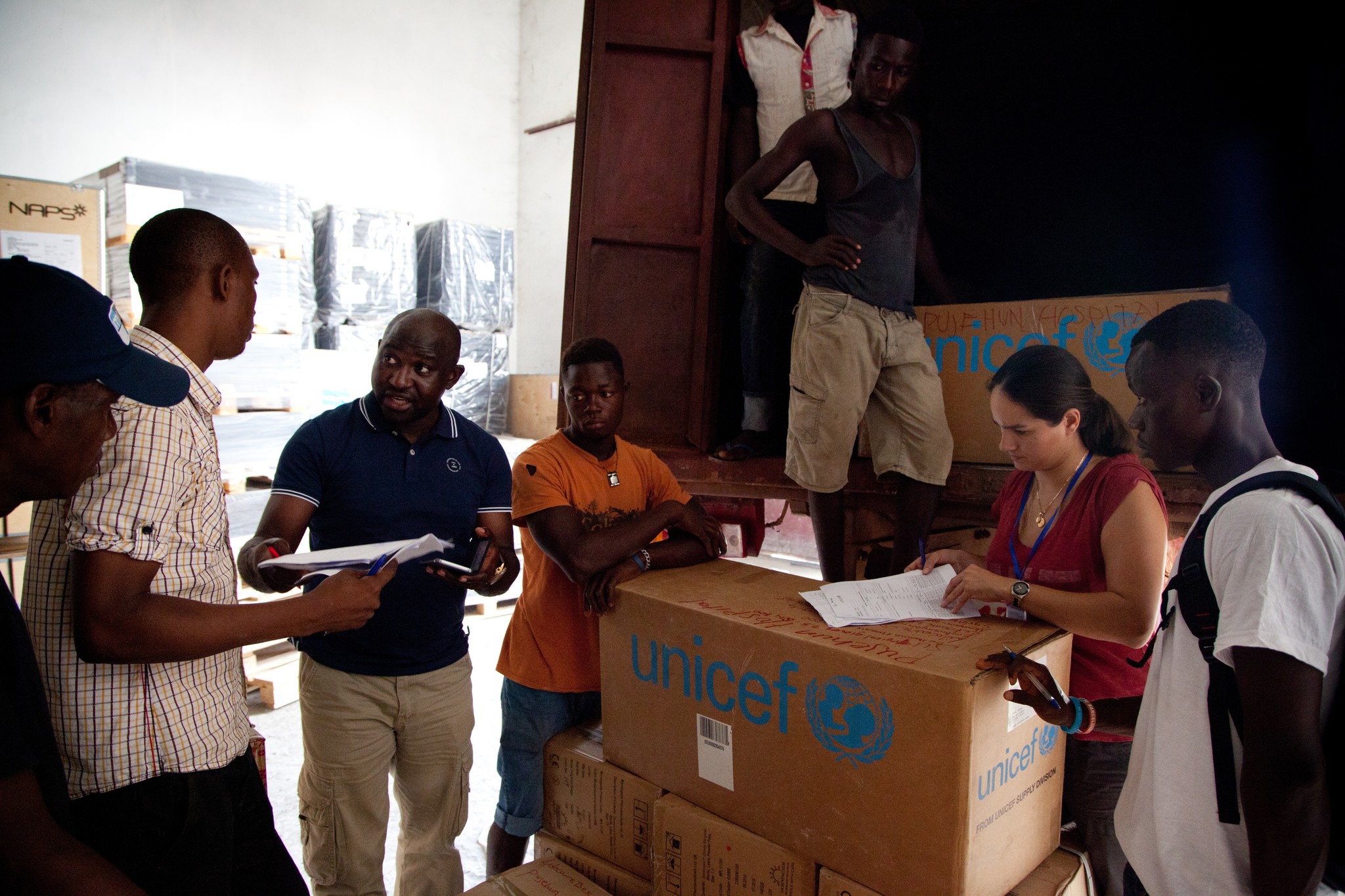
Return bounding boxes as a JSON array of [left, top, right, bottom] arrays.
[[0, 254, 191, 407]]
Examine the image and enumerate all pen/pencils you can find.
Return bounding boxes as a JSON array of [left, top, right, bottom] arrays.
[[267, 547, 301, 590], [918, 535, 926, 570], [365, 554, 387, 577], [1001, 642, 1064, 712]]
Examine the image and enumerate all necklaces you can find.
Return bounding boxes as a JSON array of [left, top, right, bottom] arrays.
[[563, 428, 621, 487], [1034, 447, 1090, 528]]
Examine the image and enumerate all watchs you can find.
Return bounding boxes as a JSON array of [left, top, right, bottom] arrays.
[[1010, 578, 1030, 610]]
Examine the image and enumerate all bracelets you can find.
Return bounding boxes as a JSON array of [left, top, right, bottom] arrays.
[[639, 548, 651, 571], [1060, 695, 1084, 734], [629, 554, 645, 573], [1078, 695, 1098, 736]]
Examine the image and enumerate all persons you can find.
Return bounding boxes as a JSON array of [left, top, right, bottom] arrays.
[[898, 345, 1173, 896], [975, 298, 1345, 896], [705, 0, 880, 461], [19, 201, 397, 896], [725, 20, 956, 587], [238, 307, 519, 896], [483, 335, 730, 875], [0, 250, 195, 896]]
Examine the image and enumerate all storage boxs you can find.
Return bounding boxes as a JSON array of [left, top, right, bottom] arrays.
[[537, 714, 1099, 896], [598, 560, 1074, 896], [859, 284, 1231, 472], [457, 859, 611, 895]]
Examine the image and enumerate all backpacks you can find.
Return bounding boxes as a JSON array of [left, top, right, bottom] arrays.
[[1174, 472, 1345, 894]]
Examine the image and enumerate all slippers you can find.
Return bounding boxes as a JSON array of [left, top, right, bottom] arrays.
[[708, 438, 773, 465]]
